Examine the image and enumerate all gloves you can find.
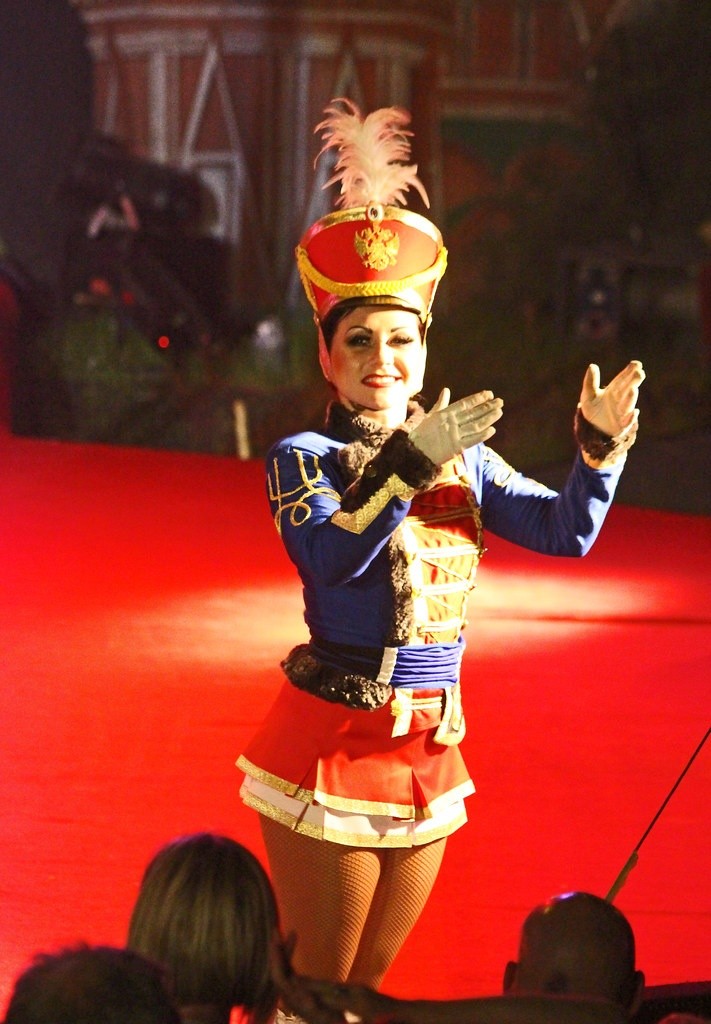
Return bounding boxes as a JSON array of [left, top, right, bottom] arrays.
[[582, 361, 645, 440], [408, 388, 503, 466]]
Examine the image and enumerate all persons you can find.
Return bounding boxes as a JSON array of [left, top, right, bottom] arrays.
[[1, 832, 711, 1024], [234, 99, 645, 1024]]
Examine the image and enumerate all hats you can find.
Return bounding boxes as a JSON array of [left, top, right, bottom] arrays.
[[295, 98, 448, 325]]
